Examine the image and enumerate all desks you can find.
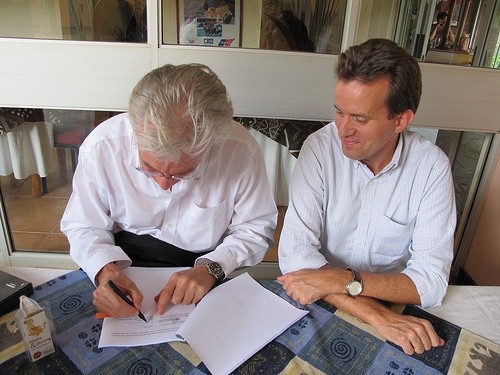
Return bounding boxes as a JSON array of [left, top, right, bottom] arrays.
[[0, 269, 500, 375], [0, 119, 55, 197]]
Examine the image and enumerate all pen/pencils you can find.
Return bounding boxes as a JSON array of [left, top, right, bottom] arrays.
[[108, 280, 149, 319]]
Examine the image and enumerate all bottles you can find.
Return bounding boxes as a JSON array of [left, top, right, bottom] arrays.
[[448, 20, 458, 49], [460, 33, 471, 52]]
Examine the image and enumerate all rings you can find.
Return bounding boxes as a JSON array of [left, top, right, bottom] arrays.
[[414, 345, 424, 352]]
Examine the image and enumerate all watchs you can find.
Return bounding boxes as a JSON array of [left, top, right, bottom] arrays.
[[202, 260, 225, 281], [346, 267, 364, 298]]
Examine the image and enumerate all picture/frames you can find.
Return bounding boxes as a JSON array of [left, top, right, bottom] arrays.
[[176, 0, 242, 48]]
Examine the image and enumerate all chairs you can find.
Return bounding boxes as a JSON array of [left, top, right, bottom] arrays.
[[43, 111, 113, 193]]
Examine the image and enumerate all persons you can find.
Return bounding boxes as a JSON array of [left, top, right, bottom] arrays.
[[59, 62, 278, 318], [431, 11, 455, 46], [277, 38, 457, 355]]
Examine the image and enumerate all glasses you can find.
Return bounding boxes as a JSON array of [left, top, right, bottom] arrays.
[[130, 129, 214, 182]]
